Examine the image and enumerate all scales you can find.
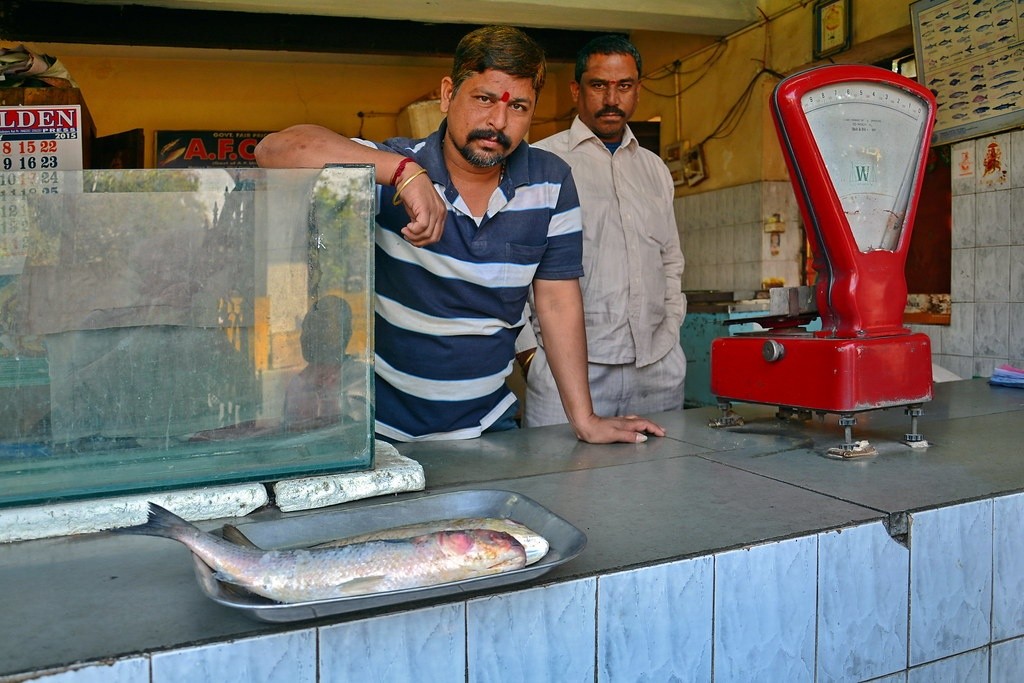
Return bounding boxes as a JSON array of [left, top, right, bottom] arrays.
[[709, 68, 937, 461]]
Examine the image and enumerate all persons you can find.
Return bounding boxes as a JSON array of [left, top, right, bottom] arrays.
[[279, 295, 367, 432], [253, 25, 668, 446], [513, 35, 689, 429]]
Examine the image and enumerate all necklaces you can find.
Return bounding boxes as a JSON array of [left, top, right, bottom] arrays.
[[440, 138, 507, 185]]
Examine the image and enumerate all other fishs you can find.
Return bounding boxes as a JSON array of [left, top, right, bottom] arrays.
[[115, 501, 550, 605]]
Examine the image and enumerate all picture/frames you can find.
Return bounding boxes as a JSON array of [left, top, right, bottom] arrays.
[[812, 0, 851, 62], [909, 0, 1024, 148]]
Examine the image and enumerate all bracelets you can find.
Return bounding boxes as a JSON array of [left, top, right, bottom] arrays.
[[392, 168, 429, 206], [520, 353, 537, 373], [388, 157, 414, 185]]
